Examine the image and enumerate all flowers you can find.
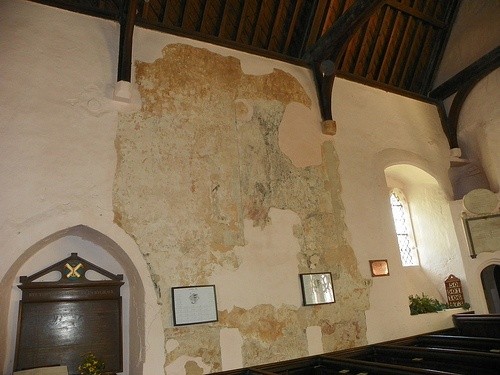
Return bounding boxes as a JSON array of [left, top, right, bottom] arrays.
[[81, 352, 104, 375]]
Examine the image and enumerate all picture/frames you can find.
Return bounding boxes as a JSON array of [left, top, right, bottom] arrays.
[[370, 259, 389, 277], [301, 272, 336, 304], [171, 285, 218, 325]]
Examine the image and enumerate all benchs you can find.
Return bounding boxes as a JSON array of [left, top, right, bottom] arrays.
[[454, 310, 500, 336], [248, 335, 499, 375]]
